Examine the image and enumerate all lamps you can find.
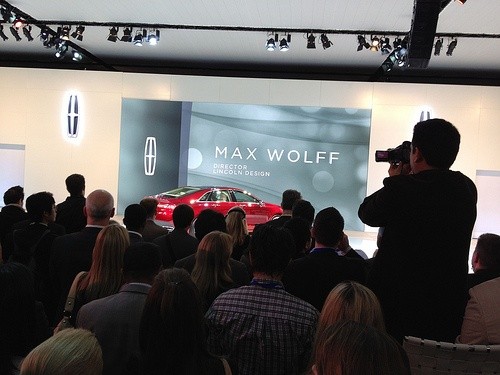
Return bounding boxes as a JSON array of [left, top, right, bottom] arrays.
[[108, 27, 118, 43], [357, 34, 458, 72], [279, 34, 292, 52], [135, 29, 147, 46], [149, 30, 160, 44], [121, 28, 133, 42], [265, 33, 279, 52], [0, 5, 85, 61], [320, 34, 334, 50], [306, 35, 317, 49]]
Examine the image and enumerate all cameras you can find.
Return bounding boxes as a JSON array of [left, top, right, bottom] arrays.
[[375, 141, 411, 164]]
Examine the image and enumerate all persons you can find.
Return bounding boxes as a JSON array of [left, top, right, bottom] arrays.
[[264, 191, 363, 310], [467, 234, 500, 289], [460, 277, 500, 345], [52, 190, 114, 272], [0, 187, 28, 263], [358, 119, 477, 348], [76, 258, 158, 375], [156, 204, 250, 273], [112, 267, 232, 375], [20, 327, 104, 375], [54, 174, 87, 233], [206, 226, 321, 375], [64, 224, 130, 326], [123, 199, 169, 256], [306, 281, 411, 375], [9, 192, 65, 263]]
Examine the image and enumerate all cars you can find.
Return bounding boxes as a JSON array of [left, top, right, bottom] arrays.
[[143, 185, 284, 239]]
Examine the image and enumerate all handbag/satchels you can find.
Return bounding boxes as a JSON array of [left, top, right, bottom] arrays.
[[54, 271, 86, 336]]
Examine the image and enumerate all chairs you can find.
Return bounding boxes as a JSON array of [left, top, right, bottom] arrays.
[[403, 335, 500, 375]]
